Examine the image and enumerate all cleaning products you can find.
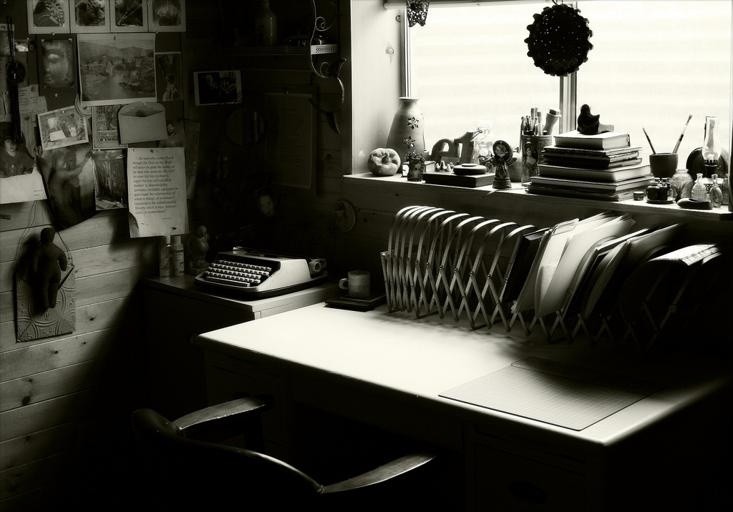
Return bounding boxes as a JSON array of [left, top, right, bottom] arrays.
[[172, 236, 185, 277], [159, 235, 171, 278]]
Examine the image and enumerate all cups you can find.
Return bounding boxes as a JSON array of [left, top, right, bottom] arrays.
[[308, 258, 329, 278], [339, 271, 371, 298]]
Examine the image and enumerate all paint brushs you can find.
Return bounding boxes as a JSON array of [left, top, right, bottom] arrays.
[[672, 115, 693, 154], [643, 128, 656, 155]]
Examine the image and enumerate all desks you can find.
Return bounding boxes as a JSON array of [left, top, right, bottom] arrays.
[[141, 273, 733, 512]]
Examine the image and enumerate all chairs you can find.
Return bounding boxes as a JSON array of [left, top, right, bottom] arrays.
[[127, 395, 442, 512]]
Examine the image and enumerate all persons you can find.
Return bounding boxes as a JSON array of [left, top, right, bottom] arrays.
[[48, 150, 93, 211], [42, 39, 73, 96], [1, 138, 36, 177], [17, 229, 67, 316]]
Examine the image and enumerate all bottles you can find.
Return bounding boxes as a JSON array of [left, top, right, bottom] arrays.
[[159, 234, 186, 277], [709, 174, 723, 208], [670, 168, 693, 199], [690, 173, 708, 203]]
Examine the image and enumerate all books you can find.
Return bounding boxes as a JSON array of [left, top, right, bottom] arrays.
[[530, 130, 656, 200], [515, 213, 723, 375]]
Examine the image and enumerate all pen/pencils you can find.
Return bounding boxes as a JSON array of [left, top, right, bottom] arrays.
[[520, 107, 561, 136]]
